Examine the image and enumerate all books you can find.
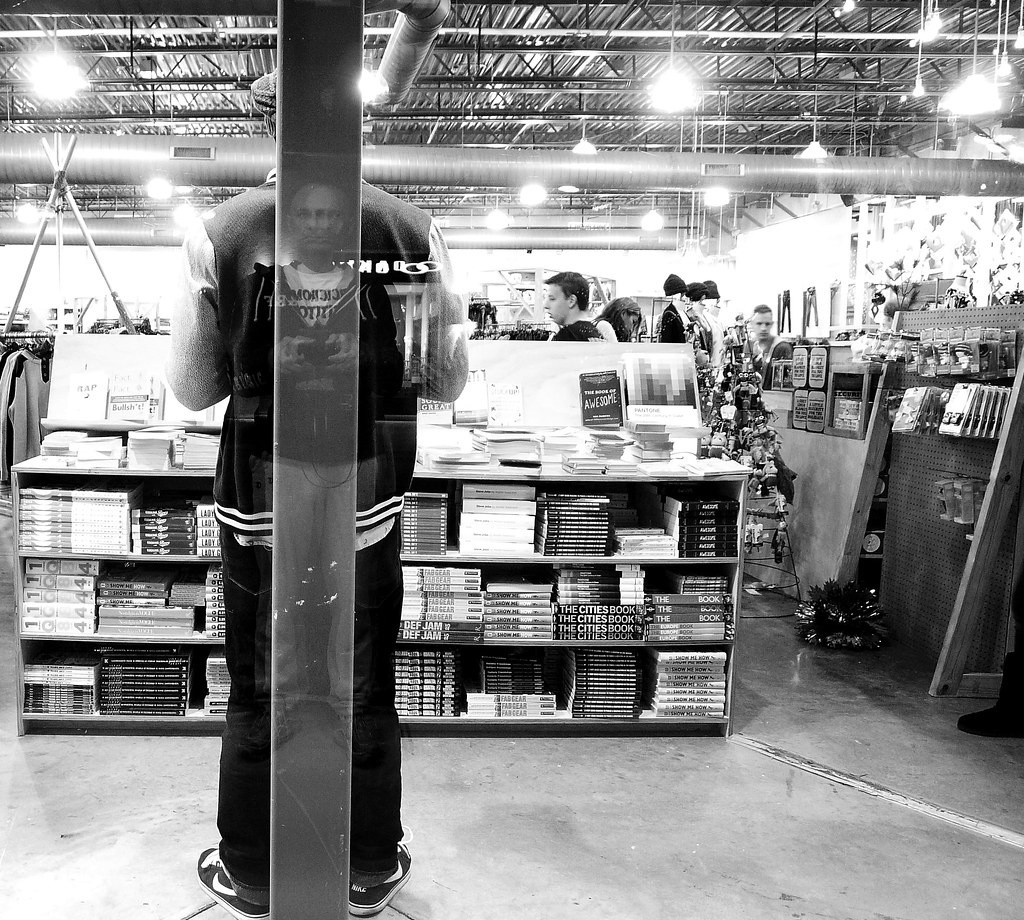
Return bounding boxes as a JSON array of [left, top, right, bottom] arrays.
[[40, 368, 751, 477], [17, 558, 740, 640], [17, 486, 739, 551], [22, 649, 728, 722]]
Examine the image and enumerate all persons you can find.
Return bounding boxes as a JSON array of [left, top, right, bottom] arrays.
[[543, 272, 602, 342], [592, 297, 641, 342], [748, 305, 793, 390], [169, 67, 471, 915], [656, 275, 726, 384], [223, 181, 404, 770]]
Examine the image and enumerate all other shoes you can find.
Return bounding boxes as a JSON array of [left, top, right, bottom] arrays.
[[275, 703, 291, 748], [334, 716, 351, 756]]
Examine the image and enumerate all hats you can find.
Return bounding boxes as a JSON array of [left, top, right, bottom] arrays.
[[663, 274, 688, 296], [688, 282, 703, 301], [703, 281, 720, 299]]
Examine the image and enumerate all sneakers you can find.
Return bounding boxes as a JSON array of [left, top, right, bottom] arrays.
[[349, 842, 412, 916], [197, 848, 273, 920]]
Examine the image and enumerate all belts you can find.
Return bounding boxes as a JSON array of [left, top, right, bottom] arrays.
[[279, 443, 355, 462]]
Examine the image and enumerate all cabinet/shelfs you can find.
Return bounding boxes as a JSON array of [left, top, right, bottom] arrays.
[[10, 333, 754, 740]]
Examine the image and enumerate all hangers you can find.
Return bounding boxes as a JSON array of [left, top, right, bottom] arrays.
[[0, 327, 54, 352], [469, 295, 549, 336]]
